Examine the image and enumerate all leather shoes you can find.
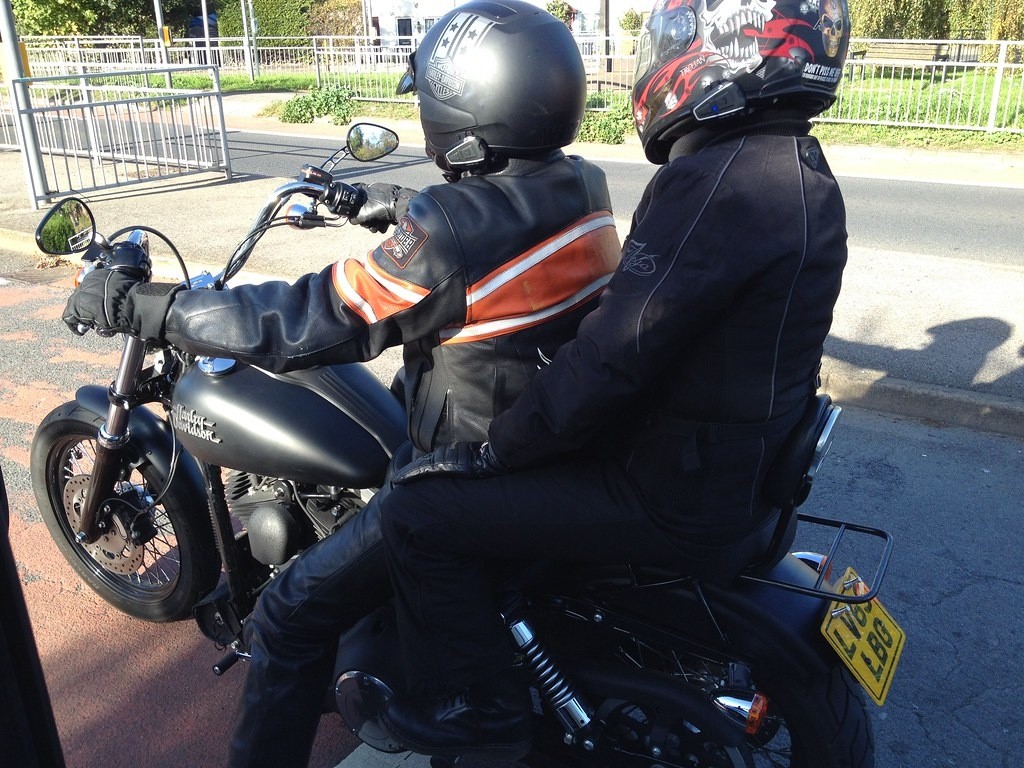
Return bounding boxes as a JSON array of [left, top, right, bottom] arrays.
[[379, 681, 536, 763]]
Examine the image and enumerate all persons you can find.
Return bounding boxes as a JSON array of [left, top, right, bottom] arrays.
[[61, 0, 619, 767], [379, 0, 854, 755]]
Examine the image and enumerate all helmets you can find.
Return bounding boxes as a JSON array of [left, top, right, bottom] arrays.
[[395, 0, 586, 184], [630, 0, 850, 166]]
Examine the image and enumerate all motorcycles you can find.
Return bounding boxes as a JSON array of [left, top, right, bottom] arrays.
[[32, 123, 906, 768]]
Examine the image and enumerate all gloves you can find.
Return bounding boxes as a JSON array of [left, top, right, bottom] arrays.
[[61, 268, 188, 350], [348, 181, 403, 235], [389, 438, 519, 485]]
